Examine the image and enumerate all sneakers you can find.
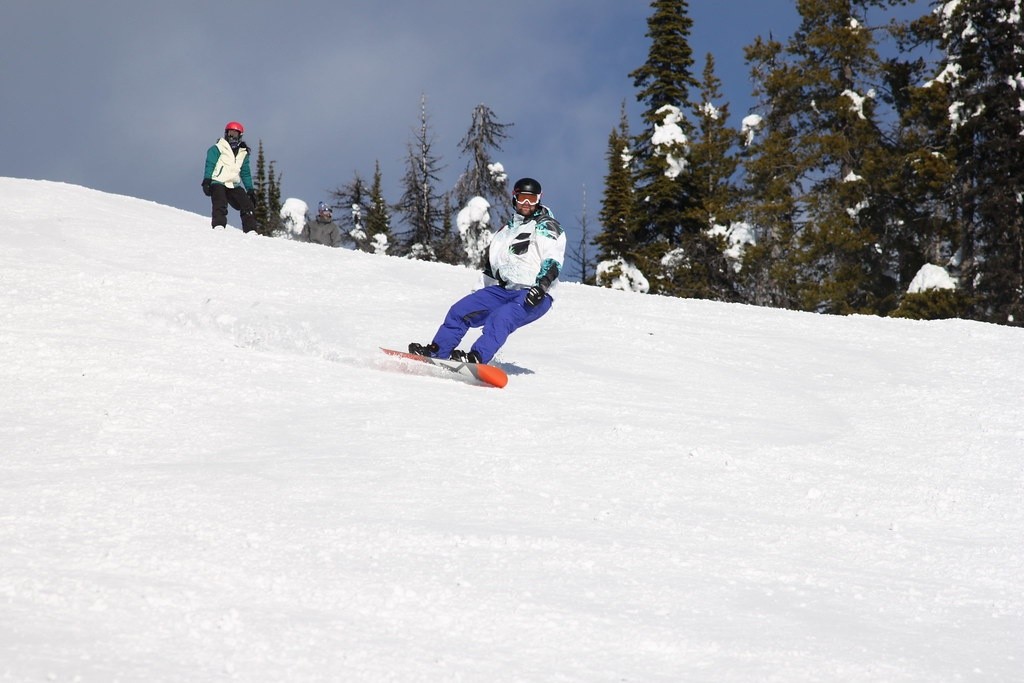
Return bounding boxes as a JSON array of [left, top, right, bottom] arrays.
[[408, 343, 438, 357], [214, 225, 225, 230], [450, 350, 482, 363], [244, 230, 258, 235]]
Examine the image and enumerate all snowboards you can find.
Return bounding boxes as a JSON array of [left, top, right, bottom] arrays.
[[370, 345, 508, 388]]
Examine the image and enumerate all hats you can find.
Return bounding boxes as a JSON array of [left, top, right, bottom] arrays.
[[318, 202, 332, 213]]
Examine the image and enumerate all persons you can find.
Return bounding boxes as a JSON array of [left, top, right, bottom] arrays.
[[301, 201, 345, 248], [201, 122, 258, 235], [408, 178, 566, 364]]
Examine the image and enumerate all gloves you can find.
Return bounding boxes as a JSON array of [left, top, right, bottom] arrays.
[[201, 179, 212, 196], [525, 286, 544, 307], [247, 190, 254, 198]]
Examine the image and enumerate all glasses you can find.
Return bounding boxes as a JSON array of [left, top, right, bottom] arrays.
[[514, 192, 541, 204]]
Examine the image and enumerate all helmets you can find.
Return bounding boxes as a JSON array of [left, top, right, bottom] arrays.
[[514, 178, 542, 195], [225, 122, 243, 134]]
